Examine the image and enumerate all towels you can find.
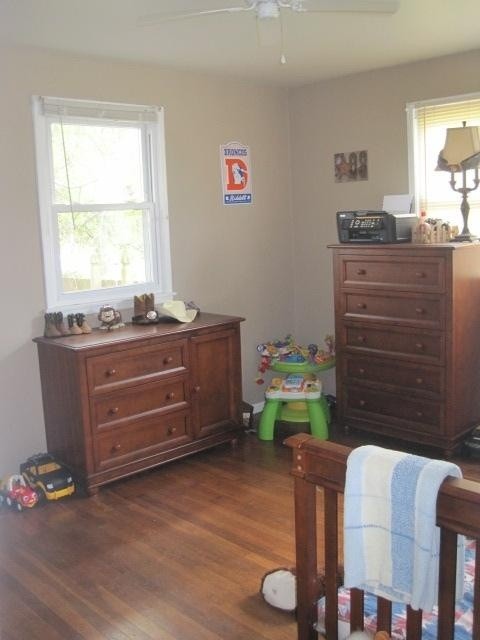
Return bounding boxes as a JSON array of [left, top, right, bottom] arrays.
[[344, 445, 464, 611]]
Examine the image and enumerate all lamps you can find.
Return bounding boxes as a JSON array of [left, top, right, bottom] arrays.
[[435, 121, 480, 242]]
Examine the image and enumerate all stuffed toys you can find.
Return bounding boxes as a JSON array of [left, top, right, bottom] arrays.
[[98, 307, 125, 329], [261, 559, 344, 611]]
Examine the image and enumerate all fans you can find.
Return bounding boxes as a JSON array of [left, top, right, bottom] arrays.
[[137, 0, 399, 64]]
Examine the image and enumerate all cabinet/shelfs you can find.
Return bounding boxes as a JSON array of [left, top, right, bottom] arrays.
[[32, 312, 246, 497], [327, 242, 480, 458]]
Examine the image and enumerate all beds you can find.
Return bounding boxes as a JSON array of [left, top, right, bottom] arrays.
[[284, 434, 480, 640]]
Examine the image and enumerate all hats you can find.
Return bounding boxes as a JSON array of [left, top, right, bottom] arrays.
[[154, 301, 198, 323]]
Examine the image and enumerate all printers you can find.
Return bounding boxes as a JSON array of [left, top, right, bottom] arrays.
[[336, 205, 419, 245]]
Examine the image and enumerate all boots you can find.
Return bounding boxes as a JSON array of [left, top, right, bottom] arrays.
[[44, 312, 92, 337], [134, 293, 159, 324]]
